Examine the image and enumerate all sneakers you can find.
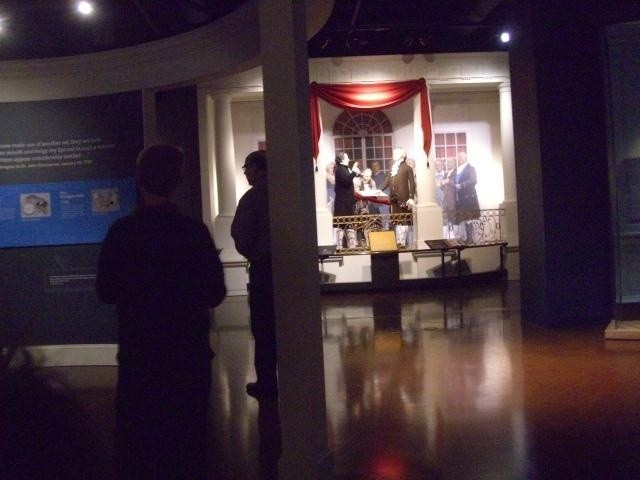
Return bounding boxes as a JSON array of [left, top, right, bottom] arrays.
[[244, 380, 277, 399]]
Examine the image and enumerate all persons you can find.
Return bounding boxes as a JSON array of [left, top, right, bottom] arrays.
[[95, 144, 227, 480], [231, 150, 276, 395], [326, 148, 481, 251]]
